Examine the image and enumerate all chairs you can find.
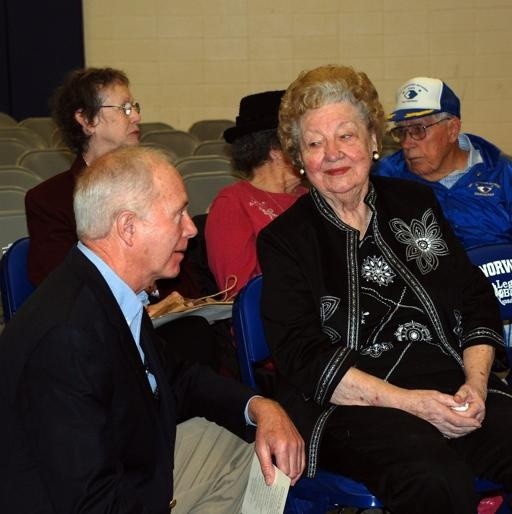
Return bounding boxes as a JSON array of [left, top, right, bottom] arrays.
[[0, 107, 511, 514]]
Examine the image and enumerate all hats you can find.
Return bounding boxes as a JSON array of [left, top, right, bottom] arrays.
[[383, 75, 464, 122], [222, 90, 289, 145]]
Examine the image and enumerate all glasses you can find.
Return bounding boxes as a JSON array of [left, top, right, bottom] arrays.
[[387, 123, 436, 143], [93, 102, 141, 116]]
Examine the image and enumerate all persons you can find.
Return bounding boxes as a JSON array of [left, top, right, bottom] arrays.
[[372, 75, 512, 343], [0, 141, 308, 514], [257, 62, 511, 513], [202, 88, 311, 307], [22, 64, 218, 296]]
